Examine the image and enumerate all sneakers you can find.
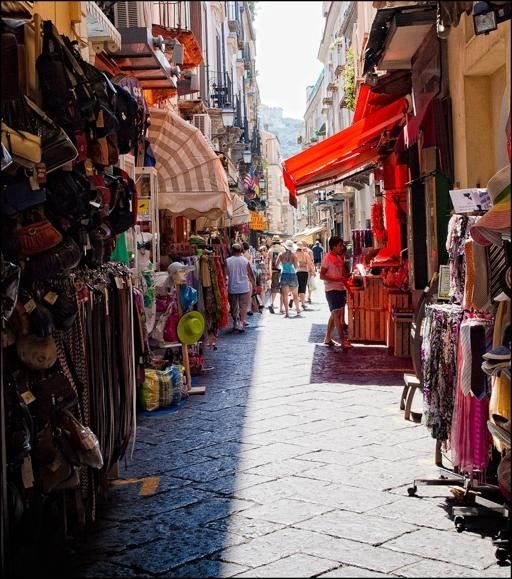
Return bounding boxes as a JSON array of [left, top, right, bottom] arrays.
[[247, 298, 311, 317], [233, 320, 250, 331]]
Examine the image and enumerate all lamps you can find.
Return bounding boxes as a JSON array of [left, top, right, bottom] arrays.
[[220, 102, 237, 129], [259, 176, 267, 201], [243, 146, 252, 164]]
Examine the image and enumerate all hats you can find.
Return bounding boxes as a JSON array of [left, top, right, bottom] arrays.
[[272, 235, 307, 253], [168, 262, 194, 276], [470, 164, 511, 246], [181, 283, 197, 311], [177, 311, 204, 344]]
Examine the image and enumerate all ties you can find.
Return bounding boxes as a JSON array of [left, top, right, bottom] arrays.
[[460, 322, 491, 401], [464, 240, 505, 310]]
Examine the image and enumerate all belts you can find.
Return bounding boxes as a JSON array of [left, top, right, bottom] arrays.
[[33, 262, 137, 531]]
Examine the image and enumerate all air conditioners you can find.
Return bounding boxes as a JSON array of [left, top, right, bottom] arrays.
[[114, 0, 152, 33], [193, 114, 212, 140]]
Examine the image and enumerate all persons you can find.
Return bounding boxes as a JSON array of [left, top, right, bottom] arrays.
[[337, 238, 357, 338], [318, 236, 357, 352], [222, 231, 324, 333]]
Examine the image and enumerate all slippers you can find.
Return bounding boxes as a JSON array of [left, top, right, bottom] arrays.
[[324, 339, 353, 348]]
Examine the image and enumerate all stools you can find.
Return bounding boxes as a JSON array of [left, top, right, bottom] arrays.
[[400, 373, 420, 421]]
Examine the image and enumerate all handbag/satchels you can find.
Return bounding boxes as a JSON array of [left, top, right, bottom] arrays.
[[0, 261, 103, 518], [142, 365, 187, 412], [1, 20, 149, 273], [189, 352, 205, 375]]
